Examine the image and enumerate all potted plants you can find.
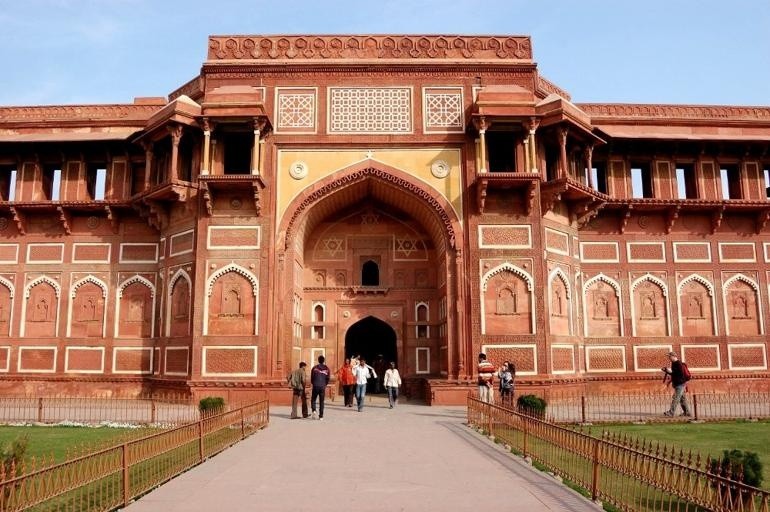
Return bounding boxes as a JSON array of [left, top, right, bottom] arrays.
[[517, 393, 547, 424], [198, 396, 224, 431], [711, 449, 762, 510]]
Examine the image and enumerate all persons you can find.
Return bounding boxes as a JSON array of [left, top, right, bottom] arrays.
[[351, 357, 373, 412], [477, 353, 495, 421], [338, 358, 355, 407], [310, 355, 331, 421], [497, 364, 516, 395], [383, 361, 403, 409], [286, 361, 309, 419], [499, 360, 516, 416], [350, 352, 361, 366], [661, 351, 692, 417]]
